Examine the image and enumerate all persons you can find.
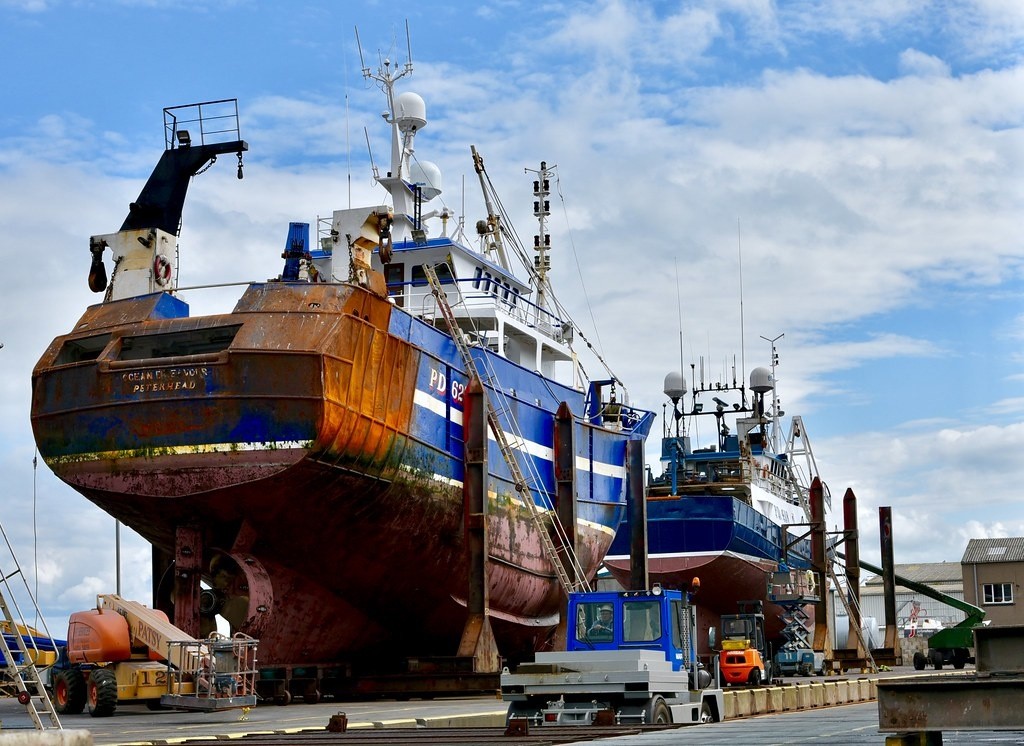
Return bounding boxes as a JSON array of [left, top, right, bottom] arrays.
[[586, 604, 614, 637]]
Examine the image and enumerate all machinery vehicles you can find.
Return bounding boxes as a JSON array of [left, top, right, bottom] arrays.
[[52, 594, 259, 718], [708, 599, 774, 688], [498, 439, 725, 726]]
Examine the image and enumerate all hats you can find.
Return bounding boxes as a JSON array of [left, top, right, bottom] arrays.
[[599, 605, 613, 611]]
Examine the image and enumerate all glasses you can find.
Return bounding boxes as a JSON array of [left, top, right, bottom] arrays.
[[602, 612, 610, 614]]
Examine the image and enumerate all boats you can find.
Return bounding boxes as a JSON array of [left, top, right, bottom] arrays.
[[28, 16, 658, 704], [603, 215, 838, 679]]
